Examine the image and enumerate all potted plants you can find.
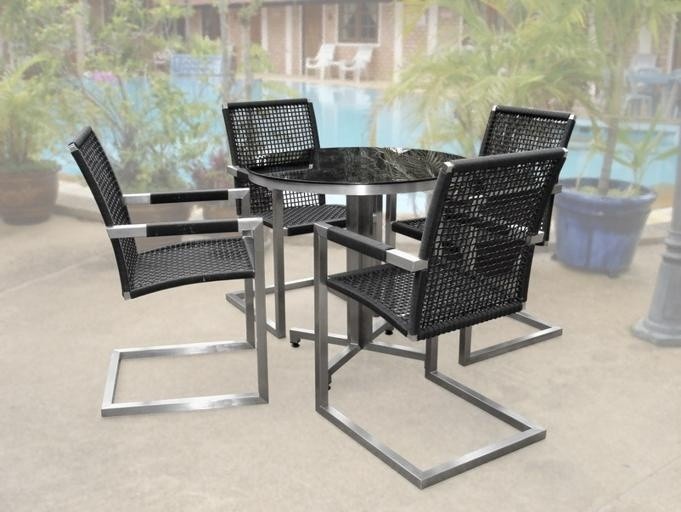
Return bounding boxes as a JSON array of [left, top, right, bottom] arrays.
[[362, 1, 681, 276]]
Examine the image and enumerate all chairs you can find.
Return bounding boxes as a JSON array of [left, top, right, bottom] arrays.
[[304, 42, 373, 85], [598, 67, 681, 119]]
